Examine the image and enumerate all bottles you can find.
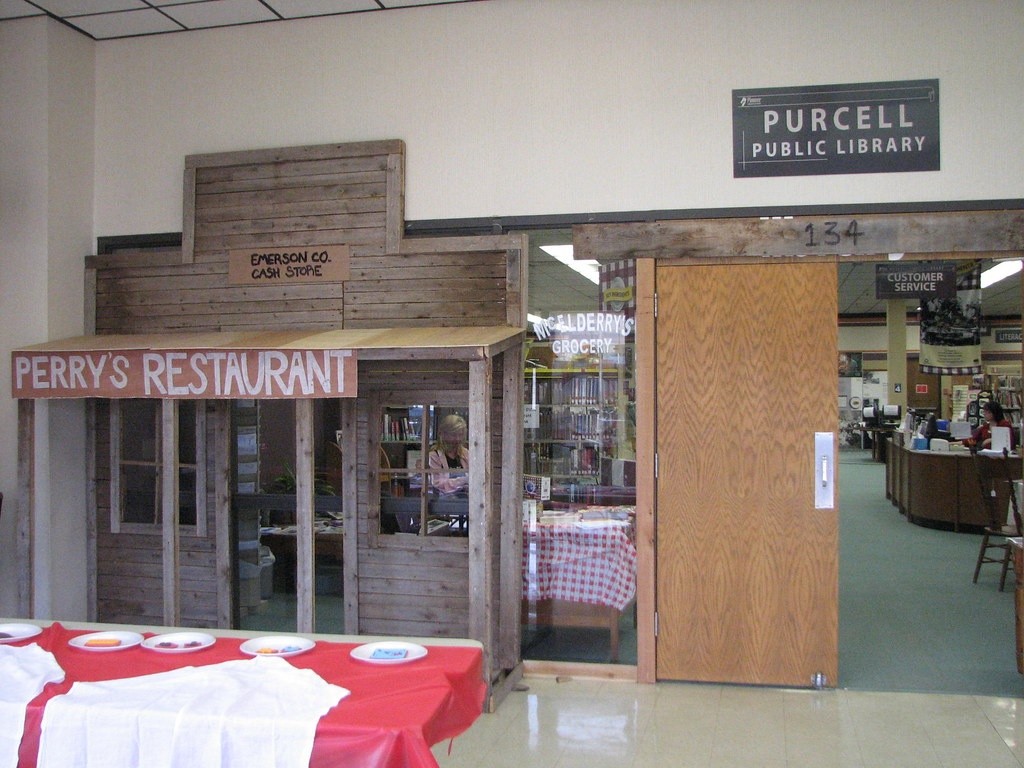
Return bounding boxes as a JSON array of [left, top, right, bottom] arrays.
[[392, 471, 400, 497]]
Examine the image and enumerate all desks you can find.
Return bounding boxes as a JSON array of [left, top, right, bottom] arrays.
[[0, 615, 489, 768], [521, 504, 637, 660], [846, 427, 892, 461]]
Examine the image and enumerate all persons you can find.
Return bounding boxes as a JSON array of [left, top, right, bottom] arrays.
[[960, 401, 1014, 452], [426, 415, 471, 496], [844, 353, 861, 377]]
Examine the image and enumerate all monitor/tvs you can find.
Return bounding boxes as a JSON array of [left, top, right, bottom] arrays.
[[882, 405, 901, 425], [863, 408, 879, 425], [925, 413, 938, 439], [907, 407, 916, 434]]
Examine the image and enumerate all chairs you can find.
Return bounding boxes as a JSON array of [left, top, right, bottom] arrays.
[[969, 445, 1024, 592]]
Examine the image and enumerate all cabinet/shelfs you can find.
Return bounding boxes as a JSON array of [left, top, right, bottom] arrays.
[[523, 369, 637, 502]]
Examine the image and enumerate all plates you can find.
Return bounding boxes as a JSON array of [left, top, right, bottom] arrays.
[[349, 641, 428, 665], [239, 635, 316, 658], [0, 623, 43, 644], [140, 632, 217, 653], [68, 630, 145, 652]]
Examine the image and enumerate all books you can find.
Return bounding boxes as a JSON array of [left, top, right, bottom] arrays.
[[522, 377, 619, 404], [523, 407, 619, 441], [406, 450, 431, 489], [997, 375, 1024, 427], [382, 410, 439, 442], [523, 446, 613, 476]]
